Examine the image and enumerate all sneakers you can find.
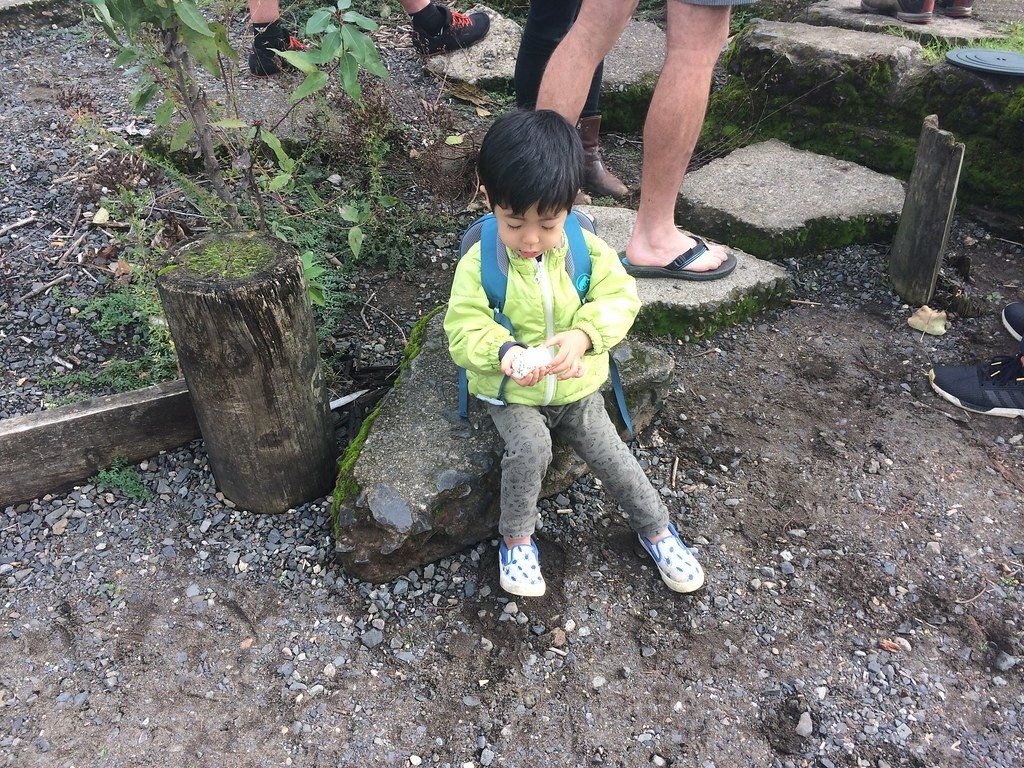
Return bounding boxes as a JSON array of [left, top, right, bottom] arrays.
[[1001, 302, 1024, 342], [249, 26, 330, 78], [929, 353, 1024, 418], [411, 4, 490, 57]]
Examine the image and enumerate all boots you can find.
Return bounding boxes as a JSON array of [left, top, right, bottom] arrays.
[[574, 189, 592, 204], [575, 115, 629, 197]]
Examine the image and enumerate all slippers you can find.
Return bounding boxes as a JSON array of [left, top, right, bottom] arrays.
[[620, 236, 737, 281], [583, 213, 598, 228]]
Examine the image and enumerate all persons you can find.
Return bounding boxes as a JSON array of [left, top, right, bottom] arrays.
[[514, 1, 627, 205], [249, 0, 491, 76], [443, 110, 704, 596], [929, 301, 1024, 417], [512, 0, 738, 278]]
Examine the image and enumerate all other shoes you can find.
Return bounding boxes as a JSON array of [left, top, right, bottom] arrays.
[[861, 0, 936, 22], [638, 521, 704, 593], [499, 536, 546, 596], [940, 0, 974, 16]]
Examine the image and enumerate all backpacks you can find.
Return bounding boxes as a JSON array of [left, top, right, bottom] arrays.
[[457, 210, 633, 430]]
